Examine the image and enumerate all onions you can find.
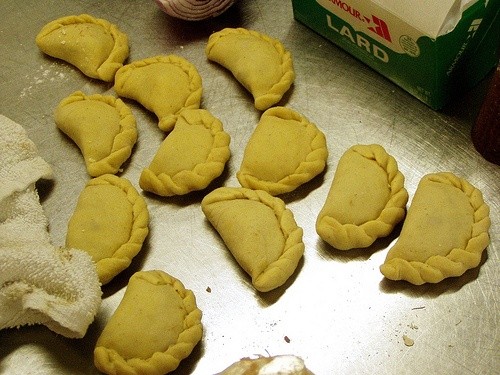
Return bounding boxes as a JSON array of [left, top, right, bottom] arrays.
[[152, 0, 236, 22]]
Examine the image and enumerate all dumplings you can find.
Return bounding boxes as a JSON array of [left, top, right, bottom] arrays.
[[92, 268, 205, 375], [237, 103, 331, 192], [314, 143, 411, 251], [380, 168, 492, 284], [35, 14, 131, 80], [201, 185, 307, 294], [206, 28, 297, 111], [112, 52, 203, 132], [65, 172, 152, 285], [139, 107, 233, 197], [55, 89, 139, 178]]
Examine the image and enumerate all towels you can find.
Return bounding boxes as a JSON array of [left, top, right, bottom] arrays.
[[0, 114, 104, 339]]
